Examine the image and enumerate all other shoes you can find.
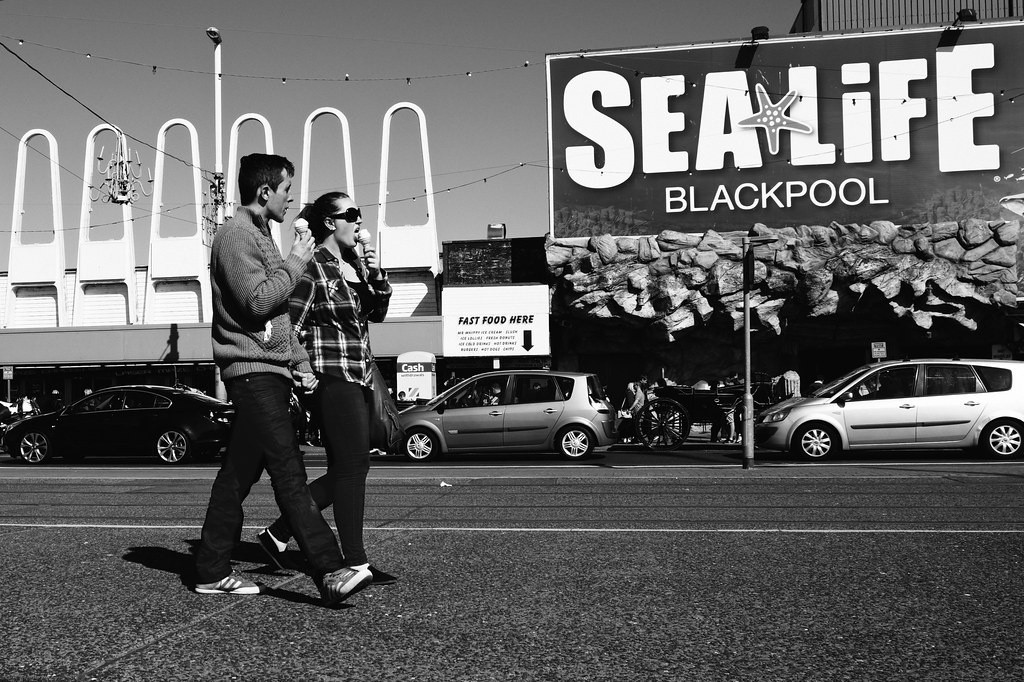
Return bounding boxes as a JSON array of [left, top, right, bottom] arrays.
[[256, 527, 290, 570], [367, 564, 398, 585], [723, 436, 736, 445]]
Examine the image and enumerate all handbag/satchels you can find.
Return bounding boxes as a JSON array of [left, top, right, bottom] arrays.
[[618, 409, 632, 419], [368, 356, 407, 454]]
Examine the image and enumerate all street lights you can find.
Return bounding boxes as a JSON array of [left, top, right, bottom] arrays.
[[206, 24, 224, 395], [740, 233, 782, 468]]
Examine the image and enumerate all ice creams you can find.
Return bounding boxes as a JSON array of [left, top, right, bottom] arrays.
[[357, 229, 372, 247], [293, 217, 307, 239]]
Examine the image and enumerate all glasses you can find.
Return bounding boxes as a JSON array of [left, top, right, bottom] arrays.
[[328, 207, 362, 223]]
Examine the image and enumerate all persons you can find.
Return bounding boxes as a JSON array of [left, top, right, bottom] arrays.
[[255, 190, 398, 587], [390, 365, 1014, 445], [94, 394, 122, 411], [194, 153, 374, 607], [84, 388, 95, 407]]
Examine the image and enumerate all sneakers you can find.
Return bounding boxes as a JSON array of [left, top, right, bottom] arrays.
[[194, 575, 260, 594], [316, 567, 373, 604]]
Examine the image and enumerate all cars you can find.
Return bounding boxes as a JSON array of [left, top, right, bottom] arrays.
[[393, 369, 615, 462], [4, 384, 237, 467], [752, 356, 1024, 461]]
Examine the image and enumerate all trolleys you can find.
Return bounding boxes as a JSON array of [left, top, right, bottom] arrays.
[[635, 380, 774, 452]]
